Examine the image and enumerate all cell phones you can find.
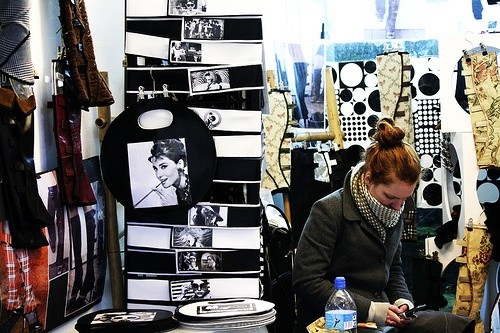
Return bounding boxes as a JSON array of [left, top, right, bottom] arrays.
[[398, 304, 426, 319]]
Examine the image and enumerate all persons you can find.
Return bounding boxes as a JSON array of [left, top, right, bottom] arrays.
[[292, 121, 476, 333], [148, 138, 190, 206], [207, 254, 221, 270], [194, 206, 224, 227], [183, 251, 196, 270], [178, 280, 211, 300]]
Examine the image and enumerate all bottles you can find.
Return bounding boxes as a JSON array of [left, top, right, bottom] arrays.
[[325, 277, 357, 333]]
